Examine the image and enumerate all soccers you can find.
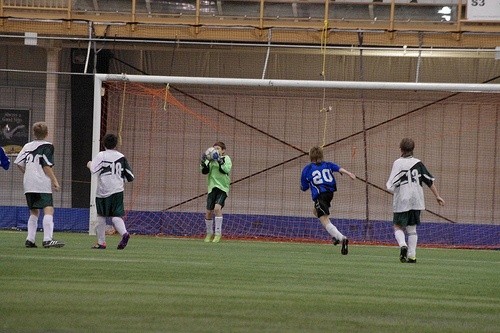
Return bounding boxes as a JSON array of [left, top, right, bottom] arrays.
[[205, 146, 218, 159]]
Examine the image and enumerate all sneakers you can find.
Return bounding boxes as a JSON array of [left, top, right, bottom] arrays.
[[340, 239, 349, 255], [42, 239, 64, 248], [212, 233, 222, 242], [332, 236, 340, 245], [204, 233, 213, 242], [117, 232, 130, 249], [25, 240, 38, 248], [407, 258, 416, 263], [91, 242, 106, 249], [400, 246, 408, 263]]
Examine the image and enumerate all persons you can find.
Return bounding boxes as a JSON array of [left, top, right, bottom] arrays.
[[14, 122, 65, 248], [0, 146, 10, 171], [388, 137, 446, 263], [300, 146, 357, 255], [86, 133, 135, 249], [200, 142, 233, 243]]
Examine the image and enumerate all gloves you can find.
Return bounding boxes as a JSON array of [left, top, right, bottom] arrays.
[[201, 153, 207, 163], [213, 152, 223, 165]]
